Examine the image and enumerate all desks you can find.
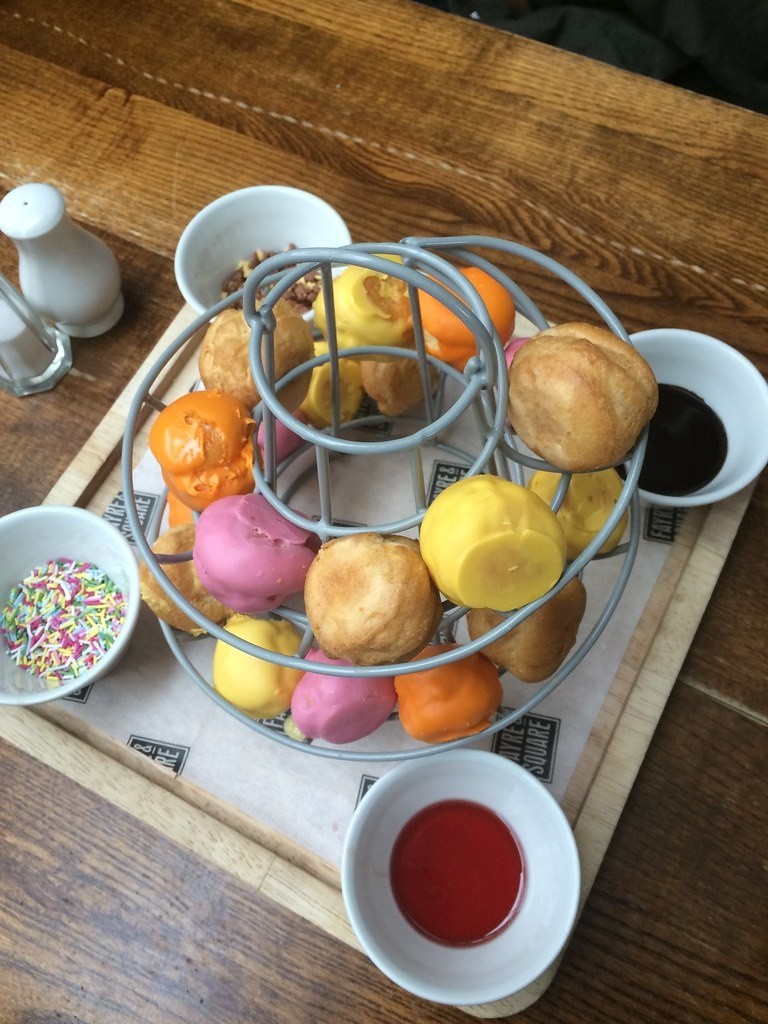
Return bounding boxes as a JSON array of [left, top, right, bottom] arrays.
[[0, 0, 768, 1022]]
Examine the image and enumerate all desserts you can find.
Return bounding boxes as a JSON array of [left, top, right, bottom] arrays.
[[140, 256, 658, 747]]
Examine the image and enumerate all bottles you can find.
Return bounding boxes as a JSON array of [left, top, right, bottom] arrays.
[[0, 183, 124, 338], [0, 271, 73, 397]]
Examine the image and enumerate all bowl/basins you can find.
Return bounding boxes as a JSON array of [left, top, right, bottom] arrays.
[[174, 184, 353, 325], [340, 748, 582, 1007], [621, 328, 768, 508], [0, 505, 141, 706]]
